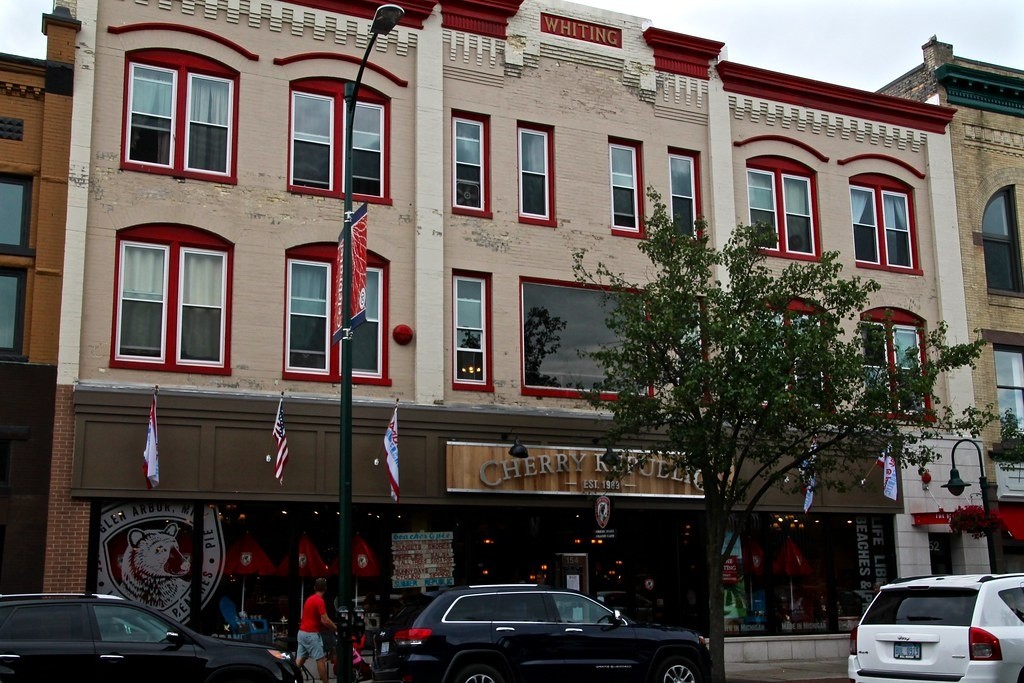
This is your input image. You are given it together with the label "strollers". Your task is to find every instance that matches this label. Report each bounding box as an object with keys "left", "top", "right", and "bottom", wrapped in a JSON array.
[{"left": 334, "top": 630, "right": 371, "bottom": 683}]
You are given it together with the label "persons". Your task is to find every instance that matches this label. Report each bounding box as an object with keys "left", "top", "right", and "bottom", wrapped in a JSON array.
[
  {"left": 331, "top": 534, "right": 380, "bottom": 607},
  {"left": 295, "top": 577, "right": 339, "bottom": 683}
]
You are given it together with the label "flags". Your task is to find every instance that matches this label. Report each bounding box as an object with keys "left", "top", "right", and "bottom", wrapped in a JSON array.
[
  {"left": 272, "top": 402, "right": 290, "bottom": 485},
  {"left": 142, "top": 397, "right": 160, "bottom": 489},
  {"left": 878, "top": 439, "right": 898, "bottom": 502},
  {"left": 798, "top": 441, "right": 819, "bottom": 517},
  {"left": 380, "top": 407, "right": 400, "bottom": 502}
]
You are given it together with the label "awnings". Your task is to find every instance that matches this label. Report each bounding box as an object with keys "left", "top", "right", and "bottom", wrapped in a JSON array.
[{"left": 986, "top": 504, "right": 1024, "bottom": 542}]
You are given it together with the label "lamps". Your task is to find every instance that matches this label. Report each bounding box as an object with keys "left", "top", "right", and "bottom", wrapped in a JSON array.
[
  {"left": 463, "top": 365, "right": 481, "bottom": 374},
  {"left": 502, "top": 428, "right": 529, "bottom": 458}
]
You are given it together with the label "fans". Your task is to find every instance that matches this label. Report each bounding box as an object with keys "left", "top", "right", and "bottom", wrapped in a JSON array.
[{"left": 456, "top": 182, "right": 480, "bottom": 208}]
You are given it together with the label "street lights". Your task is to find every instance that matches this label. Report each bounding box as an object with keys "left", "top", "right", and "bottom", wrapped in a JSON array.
[
  {"left": 339, "top": 4, "right": 406, "bottom": 622},
  {"left": 940, "top": 440, "right": 998, "bottom": 574}
]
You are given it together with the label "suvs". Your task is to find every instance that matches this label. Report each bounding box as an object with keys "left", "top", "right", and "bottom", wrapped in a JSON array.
[
  {"left": 370, "top": 584, "right": 712, "bottom": 683},
  {"left": 0, "top": 594, "right": 302, "bottom": 683},
  {"left": 846, "top": 573, "right": 1024, "bottom": 683}
]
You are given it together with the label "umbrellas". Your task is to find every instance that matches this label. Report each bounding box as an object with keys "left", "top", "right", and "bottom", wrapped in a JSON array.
[
  {"left": 274, "top": 535, "right": 332, "bottom": 620},
  {"left": 772, "top": 536, "right": 814, "bottom": 610},
  {"left": 211, "top": 533, "right": 278, "bottom": 618},
  {"left": 740, "top": 536, "right": 767, "bottom": 612}
]
